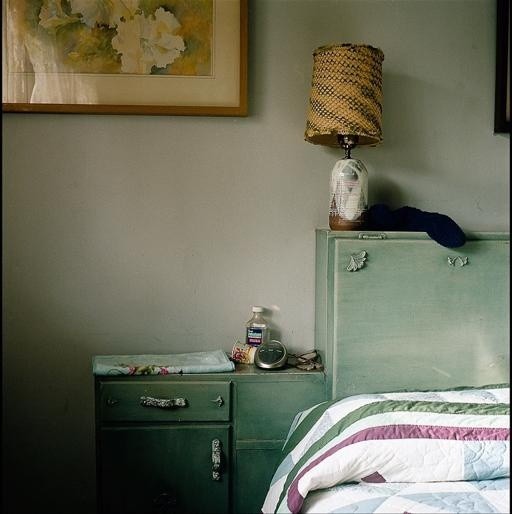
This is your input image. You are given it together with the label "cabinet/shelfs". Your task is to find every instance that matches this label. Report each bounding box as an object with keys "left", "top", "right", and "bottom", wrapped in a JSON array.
[{"left": 88, "top": 350, "right": 325, "bottom": 513}]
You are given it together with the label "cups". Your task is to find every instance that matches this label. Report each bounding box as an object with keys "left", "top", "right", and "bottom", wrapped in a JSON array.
[{"left": 231, "top": 340, "right": 256, "bottom": 365}]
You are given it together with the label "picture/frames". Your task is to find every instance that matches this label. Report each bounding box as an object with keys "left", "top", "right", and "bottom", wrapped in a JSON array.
[{"left": 1, "top": 0, "right": 249, "bottom": 118}]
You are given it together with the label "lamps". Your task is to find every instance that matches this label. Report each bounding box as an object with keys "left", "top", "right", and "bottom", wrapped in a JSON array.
[{"left": 304, "top": 41, "right": 385, "bottom": 232}]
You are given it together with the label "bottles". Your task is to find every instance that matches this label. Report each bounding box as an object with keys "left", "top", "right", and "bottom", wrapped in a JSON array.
[{"left": 246, "top": 306, "right": 270, "bottom": 347}]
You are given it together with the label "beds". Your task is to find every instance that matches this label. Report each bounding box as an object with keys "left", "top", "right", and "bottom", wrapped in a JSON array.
[{"left": 304, "top": 225, "right": 511, "bottom": 512}]
